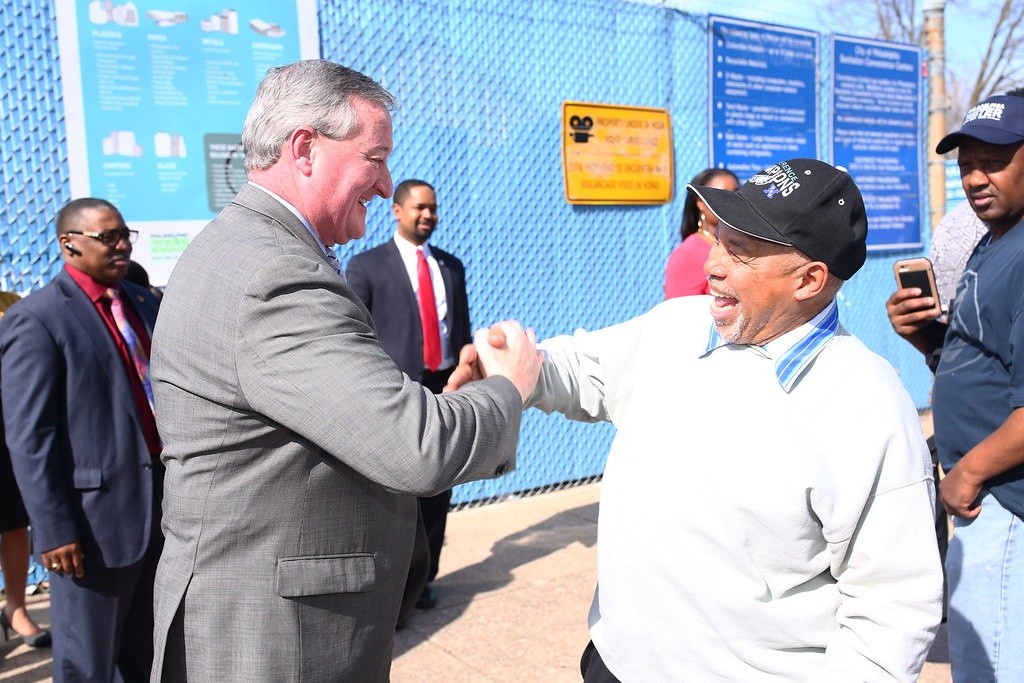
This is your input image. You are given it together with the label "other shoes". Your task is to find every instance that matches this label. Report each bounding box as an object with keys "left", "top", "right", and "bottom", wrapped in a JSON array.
[{"left": 415, "top": 582, "right": 441, "bottom": 609}]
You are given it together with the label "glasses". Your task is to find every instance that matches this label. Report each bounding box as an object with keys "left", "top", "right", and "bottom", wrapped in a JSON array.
[{"left": 64, "top": 228, "right": 139, "bottom": 247}]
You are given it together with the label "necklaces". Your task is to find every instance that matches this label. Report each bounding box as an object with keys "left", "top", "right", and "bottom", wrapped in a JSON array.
[{"left": 698, "top": 229, "right": 715, "bottom": 242}]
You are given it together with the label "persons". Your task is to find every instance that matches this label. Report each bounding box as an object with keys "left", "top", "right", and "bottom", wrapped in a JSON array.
[
  {"left": 149, "top": 61, "right": 544, "bottom": 683},
  {"left": 663, "top": 169, "right": 739, "bottom": 300},
  {"left": 345, "top": 179, "right": 472, "bottom": 610},
  {"left": 0, "top": 197, "right": 162, "bottom": 683},
  {"left": 886, "top": 88, "right": 1024, "bottom": 683},
  {"left": 444, "top": 159, "right": 944, "bottom": 683}
]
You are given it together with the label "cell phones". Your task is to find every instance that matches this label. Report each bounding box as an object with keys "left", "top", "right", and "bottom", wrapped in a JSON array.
[{"left": 894, "top": 258, "right": 942, "bottom": 322}]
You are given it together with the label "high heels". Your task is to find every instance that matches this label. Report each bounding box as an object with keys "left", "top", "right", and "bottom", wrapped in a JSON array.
[{"left": 0, "top": 607, "right": 53, "bottom": 647}]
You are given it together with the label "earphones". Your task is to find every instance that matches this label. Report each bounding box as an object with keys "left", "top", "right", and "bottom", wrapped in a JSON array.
[{"left": 65, "top": 243, "right": 81, "bottom": 257}]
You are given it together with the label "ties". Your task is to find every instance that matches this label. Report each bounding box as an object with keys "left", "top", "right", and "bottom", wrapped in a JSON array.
[
  {"left": 100, "top": 287, "right": 156, "bottom": 420},
  {"left": 416, "top": 249, "right": 443, "bottom": 373}
]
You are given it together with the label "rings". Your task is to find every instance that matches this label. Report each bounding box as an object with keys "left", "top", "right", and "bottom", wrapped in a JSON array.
[{"left": 52, "top": 563, "right": 61, "bottom": 571}]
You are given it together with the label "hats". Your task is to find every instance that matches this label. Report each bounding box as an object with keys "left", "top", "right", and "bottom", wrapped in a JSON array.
[
  {"left": 935, "top": 95, "right": 1024, "bottom": 154},
  {"left": 686, "top": 158, "right": 868, "bottom": 280}
]
